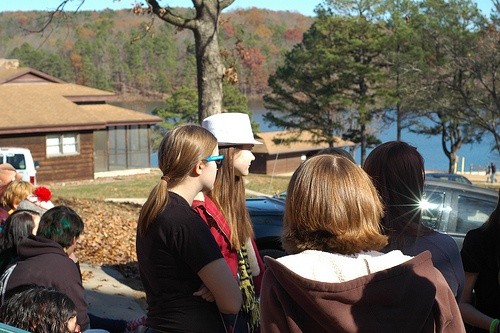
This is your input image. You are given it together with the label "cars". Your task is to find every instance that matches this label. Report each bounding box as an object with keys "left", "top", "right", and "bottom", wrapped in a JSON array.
[
  {"left": 427, "top": 173, "right": 472, "bottom": 185},
  {"left": 242, "top": 191, "right": 288, "bottom": 270},
  {"left": 421, "top": 181, "right": 499, "bottom": 255}
]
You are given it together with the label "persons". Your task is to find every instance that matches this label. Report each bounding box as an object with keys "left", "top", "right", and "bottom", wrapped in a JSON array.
[
  {"left": 0, "top": 112, "right": 500, "bottom": 333},
  {"left": 260, "top": 154, "right": 465, "bottom": 333}
]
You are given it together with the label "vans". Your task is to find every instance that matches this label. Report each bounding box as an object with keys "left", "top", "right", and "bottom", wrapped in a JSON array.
[{"left": 0, "top": 146, "right": 39, "bottom": 186}]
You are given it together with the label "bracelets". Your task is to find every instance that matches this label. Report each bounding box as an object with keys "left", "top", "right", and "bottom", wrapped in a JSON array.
[{"left": 490, "top": 319, "right": 500, "bottom": 332}]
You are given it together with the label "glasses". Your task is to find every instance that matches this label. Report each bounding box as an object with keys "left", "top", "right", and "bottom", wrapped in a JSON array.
[{"left": 201, "top": 154, "right": 224, "bottom": 168}]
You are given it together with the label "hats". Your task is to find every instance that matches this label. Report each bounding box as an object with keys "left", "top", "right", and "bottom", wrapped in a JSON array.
[
  {"left": 17, "top": 186, "right": 55, "bottom": 217},
  {"left": 202, "top": 112, "right": 263, "bottom": 145}
]
